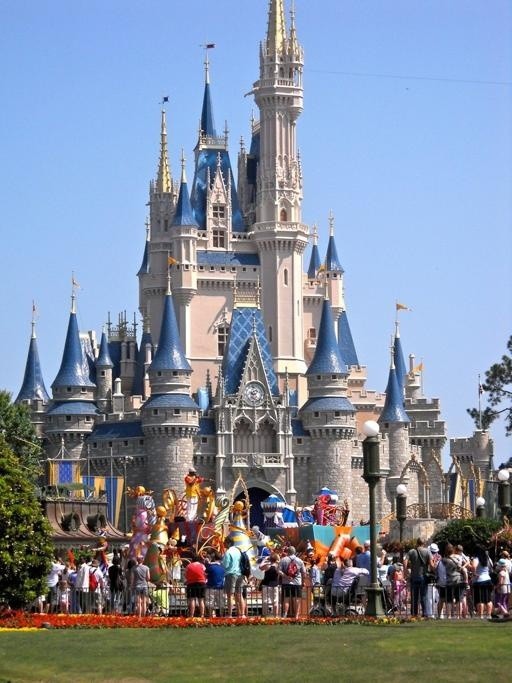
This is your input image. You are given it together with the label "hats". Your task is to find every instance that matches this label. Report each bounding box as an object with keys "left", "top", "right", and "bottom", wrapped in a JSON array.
[
  {"left": 427, "top": 543, "right": 440, "bottom": 553},
  {"left": 88, "top": 560, "right": 100, "bottom": 566}
]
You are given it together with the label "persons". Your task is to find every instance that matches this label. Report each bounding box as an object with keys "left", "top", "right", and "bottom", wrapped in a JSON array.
[{"left": 29, "top": 537, "right": 511, "bottom": 620}]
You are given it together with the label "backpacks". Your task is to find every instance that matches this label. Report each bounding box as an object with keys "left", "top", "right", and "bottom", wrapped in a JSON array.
[
  {"left": 235, "top": 546, "right": 250, "bottom": 578},
  {"left": 286, "top": 556, "right": 299, "bottom": 577},
  {"left": 89, "top": 569, "right": 98, "bottom": 591}
]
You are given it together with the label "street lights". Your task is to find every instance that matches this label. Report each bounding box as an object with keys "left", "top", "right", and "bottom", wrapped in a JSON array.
[
  {"left": 360, "top": 420, "right": 407, "bottom": 618},
  {"left": 498, "top": 470, "right": 510, "bottom": 528},
  {"left": 117, "top": 455, "right": 133, "bottom": 533},
  {"left": 476, "top": 497, "right": 485, "bottom": 517}
]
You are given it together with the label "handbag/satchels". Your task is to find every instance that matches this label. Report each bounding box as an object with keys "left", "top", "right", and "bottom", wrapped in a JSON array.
[
  {"left": 489, "top": 570, "right": 500, "bottom": 586},
  {"left": 116, "top": 575, "right": 128, "bottom": 593}
]
